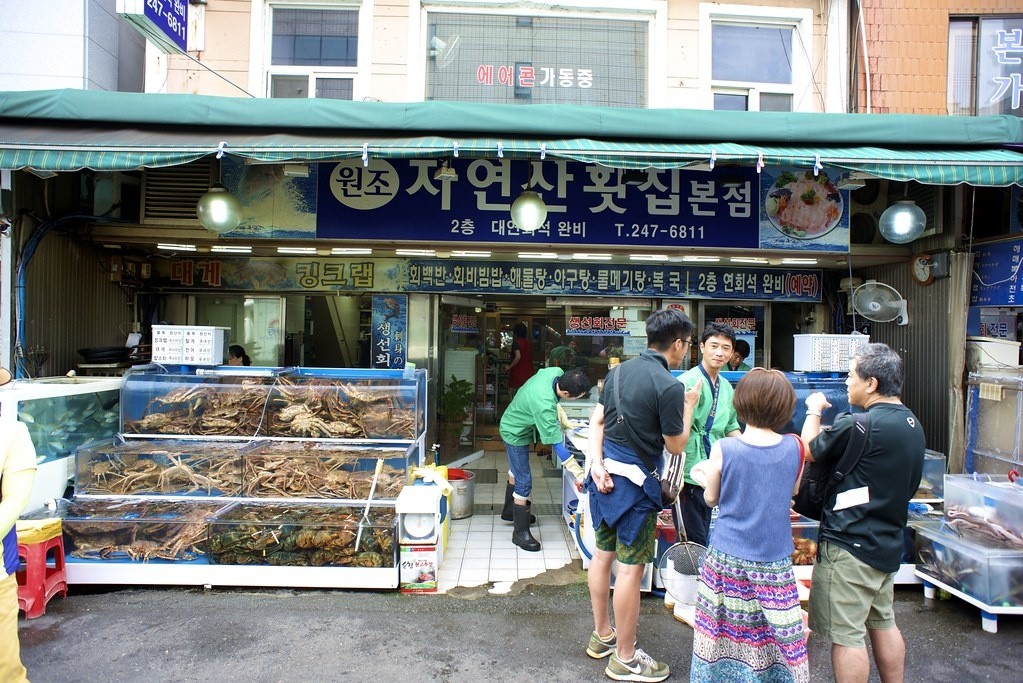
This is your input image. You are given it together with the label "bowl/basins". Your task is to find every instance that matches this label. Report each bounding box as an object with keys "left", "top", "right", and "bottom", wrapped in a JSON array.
[{"left": 404, "top": 513, "right": 435, "bottom": 538}]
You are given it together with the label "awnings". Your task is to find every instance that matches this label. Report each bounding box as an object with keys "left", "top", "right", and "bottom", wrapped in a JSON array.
[{"left": 0, "top": 91, "right": 1023, "bottom": 187}]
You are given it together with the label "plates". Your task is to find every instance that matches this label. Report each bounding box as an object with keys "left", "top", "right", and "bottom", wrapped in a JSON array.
[{"left": 765, "top": 172, "right": 843, "bottom": 239}]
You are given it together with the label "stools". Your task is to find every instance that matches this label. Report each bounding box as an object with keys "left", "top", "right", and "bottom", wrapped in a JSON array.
[{"left": 15, "top": 518, "right": 68, "bottom": 621}]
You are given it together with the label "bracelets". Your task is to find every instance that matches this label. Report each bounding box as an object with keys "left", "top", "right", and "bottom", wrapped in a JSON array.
[{"left": 805, "top": 410, "right": 823, "bottom": 417}]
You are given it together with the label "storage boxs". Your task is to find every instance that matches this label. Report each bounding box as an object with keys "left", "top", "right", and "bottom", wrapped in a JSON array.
[
  {"left": 943, "top": 474, "right": 1023, "bottom": 539},
  {"left": 790, "top": 515, "right": 821, "bottom": 566},
  {"left": 908, "top": 449, "right": 947, "bottom": 503},
  {"left": 911, "top": 521, "right": 1023, "bottom": 607},
  {"left": 966, "top": 336, "right": 1021, "bottom": 371},
  {"left": 19, "top": 369, "right": 427, "bottom": 569}
]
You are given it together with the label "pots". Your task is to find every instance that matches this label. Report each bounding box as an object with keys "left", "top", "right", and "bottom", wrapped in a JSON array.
[{"left": 77, "top": 344, "right": 152, "bottom": 364}]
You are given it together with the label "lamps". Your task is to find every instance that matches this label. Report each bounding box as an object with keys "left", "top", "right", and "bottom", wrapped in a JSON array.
[
  {"left": 196, "top": 157, "right": 244, "bottom": 235},
  {"left": 434, "top": 157, "right": 456, "bottom": 181},
  {"left": 879, "top": 181, "right": 926, "bottom": 244},
  {"left": 510, "top": 161, "right": 547, "bottom": 231},
  {"left": 836, "top": 171, "right": 866, "bottom": 190},
  {"left": 282, "top": 164, "right": 312, "bottom": 178}
]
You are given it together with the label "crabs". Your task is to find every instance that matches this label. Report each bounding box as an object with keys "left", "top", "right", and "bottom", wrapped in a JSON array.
[{"left": 55, "top": 370, "right": 422, "bottom": 570}]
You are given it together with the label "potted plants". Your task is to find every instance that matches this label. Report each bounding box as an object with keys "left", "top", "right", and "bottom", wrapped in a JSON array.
[{"left": 437, "top": 374, "right": 483, "bottom": 464}]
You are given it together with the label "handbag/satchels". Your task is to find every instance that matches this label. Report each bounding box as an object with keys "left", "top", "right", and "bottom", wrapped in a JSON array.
[{"left": 659, "top": 444, "right": 686, "bottom": 508}]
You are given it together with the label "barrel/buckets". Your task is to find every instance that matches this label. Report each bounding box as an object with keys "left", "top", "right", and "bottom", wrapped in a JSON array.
[{"left": 448, "top": 469, "right": 476, "bottom": 519}]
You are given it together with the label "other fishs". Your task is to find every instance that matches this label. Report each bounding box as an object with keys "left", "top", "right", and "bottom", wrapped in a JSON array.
[{"left": 919, "top": 499, "right": 1023, "bottom": 606}]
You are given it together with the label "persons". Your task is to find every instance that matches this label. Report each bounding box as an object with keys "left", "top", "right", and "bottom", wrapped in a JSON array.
[
  {"left": 793, "top": 344, "right": 926, "bottom": 683},
  {"left": 721, "top": 338, "right": 751, "bottom": 371},
  {"left": 0, "top": 365, "right": 39, "bottom": 683},
  {"left": 501, "top": 367, "right": 585, "bottom": 552},
  {"left": 227, "top": 343, "right": 252, "bottom": 369},
  {"left": 502, "top": 323, "right": 534, "bottom": 405},
  {"left": 664, "top": 322, "right": 743, "bottom": 630},
  {"left": 689, "top": 370, "right": 811, "bottom": 683},
  {"left": 586, "top": 308, "right": 703, "bottom": 683},
  {"left": 551, "top": 325, "right": 585, "bottom": 365}
]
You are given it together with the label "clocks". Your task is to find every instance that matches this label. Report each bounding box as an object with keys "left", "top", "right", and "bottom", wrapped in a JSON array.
[{"left": 910, "top": 254, "right": 935, "bottom": 287}]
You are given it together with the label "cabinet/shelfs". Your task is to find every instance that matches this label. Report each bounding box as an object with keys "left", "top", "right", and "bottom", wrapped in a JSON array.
[{"left": 476, "top": 358, "right": 545, "bottom": 423}]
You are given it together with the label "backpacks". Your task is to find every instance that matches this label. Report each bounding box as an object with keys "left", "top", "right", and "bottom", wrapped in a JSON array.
[{"left": 793, "top": 411, "right": 870, "bottom": 563}]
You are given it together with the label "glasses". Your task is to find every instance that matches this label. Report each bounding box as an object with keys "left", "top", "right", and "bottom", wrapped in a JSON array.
[
  {"left": 671, "top": 337, "right": 692, "bottom": 348},
  {"left": 746, "top": 366, "right": 785, "bottom": 379}
]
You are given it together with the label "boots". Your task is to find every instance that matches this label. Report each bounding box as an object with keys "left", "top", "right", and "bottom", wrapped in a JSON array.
[
  {"left": 500, "top": 480, "right": 536, "bottom": 524},
  {"left": 512, "top": 500, "right": 541, "bottom": 552},
  {"left": 664, "top": 556, "right": 676, "bottom": 608},
  {"left": 673, "top": 568, "right": 701, "bottom": 629}
]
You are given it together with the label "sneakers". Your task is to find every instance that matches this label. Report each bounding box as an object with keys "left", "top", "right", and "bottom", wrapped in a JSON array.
[
  {"left": 587, "top": 627, "right": 638, "bottom": 659},
  {"left": 605, "top": 649, "right": 670, "bottom": 682}
]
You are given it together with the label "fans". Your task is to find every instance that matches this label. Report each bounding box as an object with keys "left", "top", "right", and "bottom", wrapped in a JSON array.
[
  {"left": 429, "top": 34, "right": 461, "bottom": 69},
  {"left": 853, "top": 281, "right": 908, "bottom": 326}
]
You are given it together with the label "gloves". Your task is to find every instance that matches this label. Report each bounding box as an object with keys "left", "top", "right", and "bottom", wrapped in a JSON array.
[
  {"left": 561, "top": 454, "right": 585, "bottom": 483},
  {"left": 556, "top": 402, "right": 576, "bottom": 429}
]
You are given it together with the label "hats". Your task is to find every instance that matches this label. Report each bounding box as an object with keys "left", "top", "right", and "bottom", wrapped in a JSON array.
[{"left": 505, "top": 321, "right": 527, "bottom": 336}]
